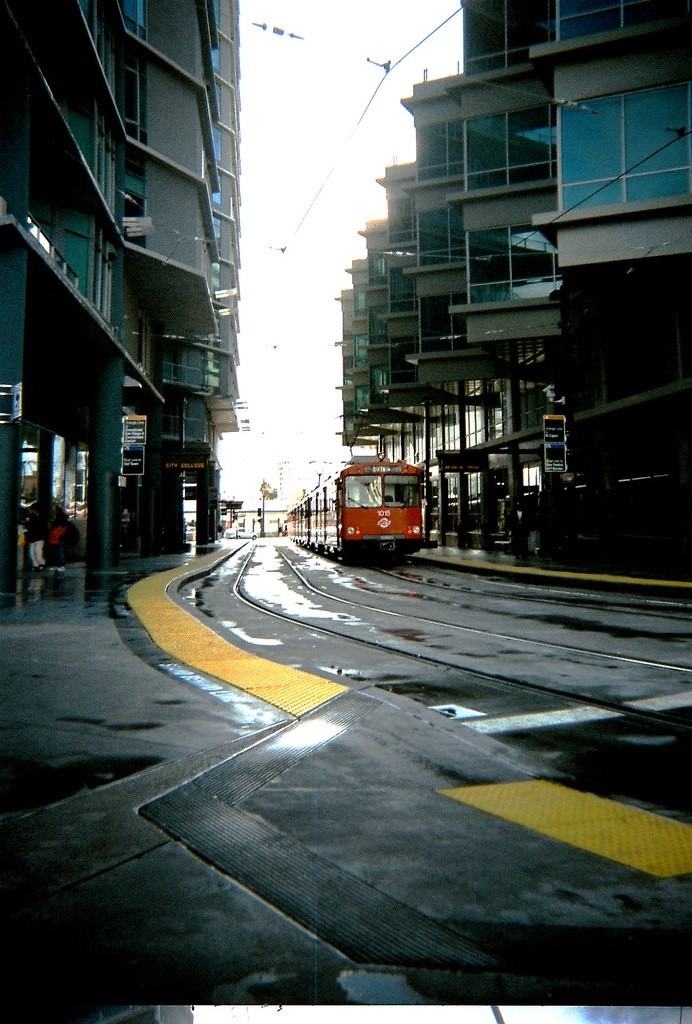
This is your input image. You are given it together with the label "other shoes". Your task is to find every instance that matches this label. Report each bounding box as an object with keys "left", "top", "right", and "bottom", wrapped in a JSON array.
[
  {"left": 58, "top": 567, "right": 65, "bottom": 572},
  {"left": 49, "top": 566, "right": 58, "bottom": 571},
  {"left": 32, "top": 565, "right": 45, "bottom": 571}
]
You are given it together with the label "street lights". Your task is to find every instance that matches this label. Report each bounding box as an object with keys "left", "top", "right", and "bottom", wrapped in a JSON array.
[{"left": 259, "top": 494, "right": 268, "bottom": 537}]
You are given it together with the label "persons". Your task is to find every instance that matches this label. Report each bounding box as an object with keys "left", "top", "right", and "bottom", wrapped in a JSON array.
[
  {"left": 236, "top": 527, "right": 239, "bottom": 539},
  {"left": 217, "top": 522, "right": 223, "bottom": 542},
  {"left": 278, "top": 525, "right": 288, "bottom": 538},
  {"left": 184, "top": 525, "right": 187, "bottom": 542},
  {"left": 24, "top": 502, "right": 72, "bottom": 572},
  {"left": 505, "top": 501, "right": 559, "bottom": 562}
]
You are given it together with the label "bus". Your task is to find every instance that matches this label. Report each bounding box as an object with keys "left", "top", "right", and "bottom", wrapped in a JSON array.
[{"left": 284, "top": 412, "right": 425, "bottom": 564}]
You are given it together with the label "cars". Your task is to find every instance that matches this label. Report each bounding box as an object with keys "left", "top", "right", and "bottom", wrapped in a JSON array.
[{"left": 224, "top": 526, "right": 258, "bottom": 540}]
